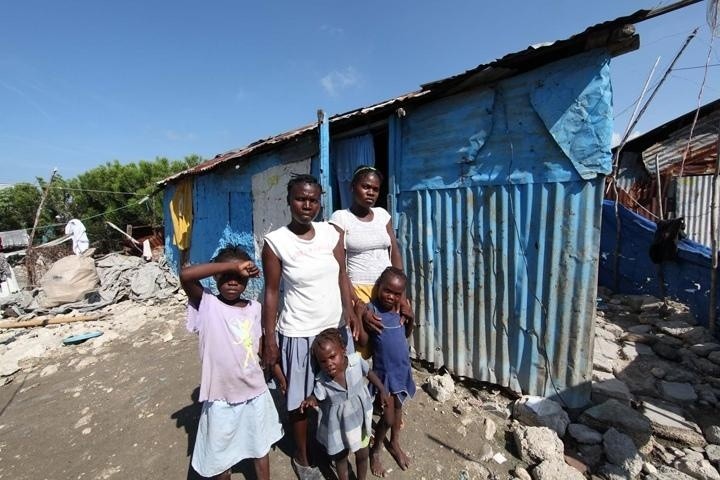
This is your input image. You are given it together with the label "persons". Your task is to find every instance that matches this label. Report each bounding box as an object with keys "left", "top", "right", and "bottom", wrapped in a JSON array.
[
  {"left": 299, "top": 328, "right": 391, "bottom": 480},
  {"left": 327, "top": 165, "right": 409, "bottom": 447},
  {"left": 180, "top": 243, "right": 287, "bottom": 479},
  {"left": 354, "top": 267, "right": 415, "bottom": 477},
  {"left": 260, "top": 174, "right": 362, "bottom": 479}
]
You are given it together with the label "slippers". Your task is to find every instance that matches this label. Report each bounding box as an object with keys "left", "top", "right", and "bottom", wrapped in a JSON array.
[{"left": 293, "top": 458, "right": 326, "bottom": 480}]
